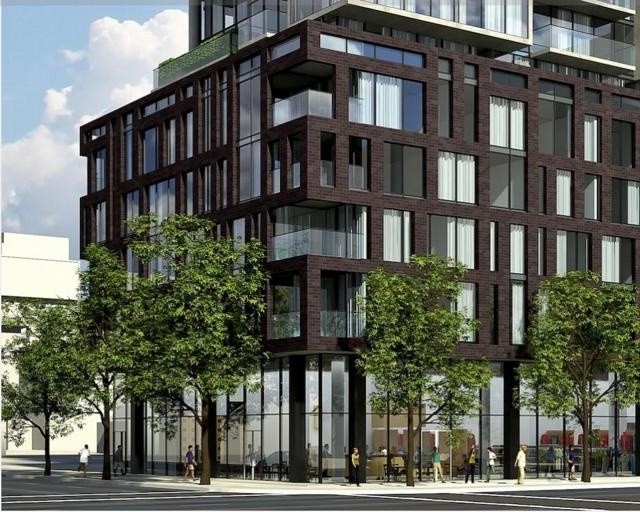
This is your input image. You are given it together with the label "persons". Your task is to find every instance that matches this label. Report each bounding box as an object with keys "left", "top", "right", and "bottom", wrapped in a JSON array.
[
  {"left": 431, "top": 446, "right": 446, "bottom": 483},
  {"left": 182, "top": 443, "right": 200, "bottom": 481},
  {"left": 567, "top": 443, "right": 577, "bottom": 480},
  {"left": 113, "top": 445, "right": 128, "bottom": 475},
  {"left": 514, "top": 444, "right": 529, "bottom": 484},
  {"left": 245, "top": 443, "right": 258, "bottom": 479},
  {"left": 465, "top": 444, "right": 478, "bottom": 483},
  {"left": 77, "top": 443, "right": 90, "bottom": 477},
  {"left": 322, "top": 443, "right": 333, "bottom": 477},
  {"left": 305, "top": 443, "right": 314, "bottom": 480},
  {"left": 484, "top": 446, "right": 497, "bottom": 482},
  {"left": 543, "top": 445, "right": 557, "bottom": 478},
  {"left": 348, "top": 447, "right": 360, "bottom": 486},
  {"left": 366, "top": 444, "right": 422, "bottom": 480},
  {"left": 457, "top": 453, "right": 470, "bottom": 473},
  {"left": 194, "top": 443, "right": 202, "bottom": 480}
]
